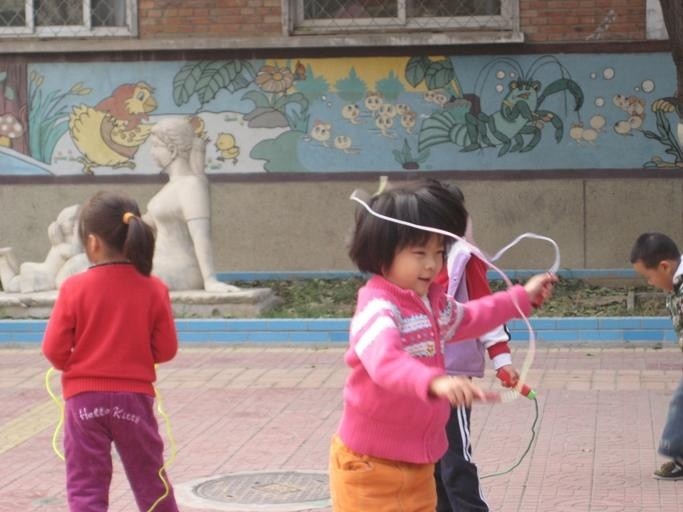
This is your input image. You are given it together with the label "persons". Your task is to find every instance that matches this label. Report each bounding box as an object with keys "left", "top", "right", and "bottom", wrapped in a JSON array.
[
  {"left": 328, "top": 190, "right": 561, "bottom": 512},
  {"left": 0, "top": 109, "right": 240, "bottom": 293},
  {"left": 628, "top": 232, "right": 683, "bottom": 481},
  {"left": 41, "top": 191, "right": 183, "bottom": 512},
  {"left": 10, "top": 203, "right": 85, "bottom": 293},
  {"left": 415, "top": 174, "right": 525, "bottom": 512}
]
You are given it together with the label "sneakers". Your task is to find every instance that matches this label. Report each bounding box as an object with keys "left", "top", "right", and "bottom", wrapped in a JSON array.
[{"left": 652, "top": 460, "right": 683, "bottom": 481}]
